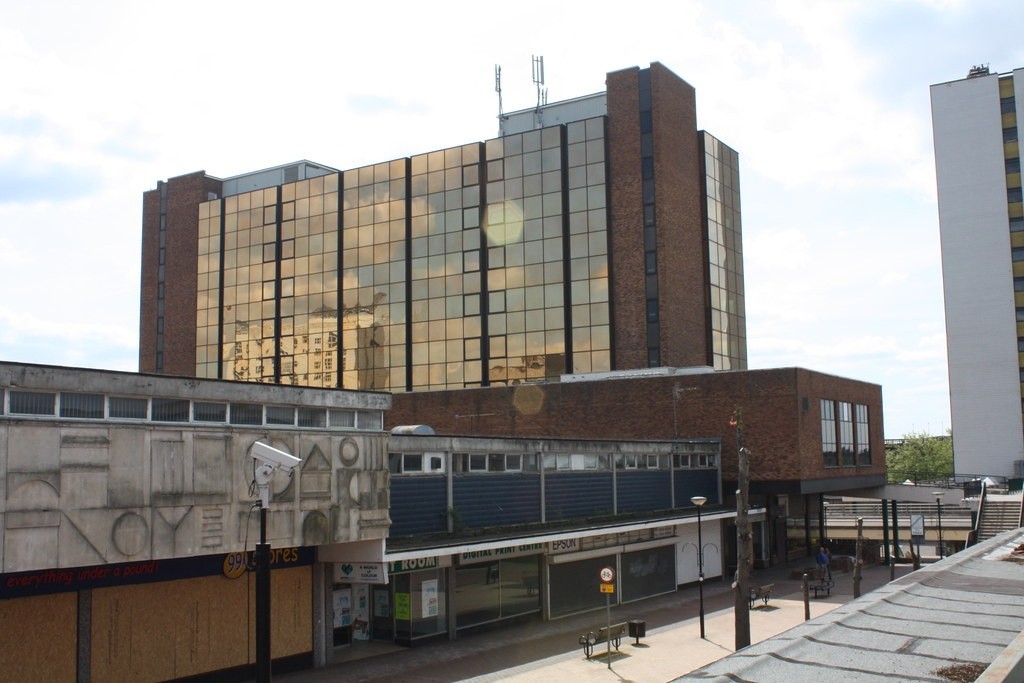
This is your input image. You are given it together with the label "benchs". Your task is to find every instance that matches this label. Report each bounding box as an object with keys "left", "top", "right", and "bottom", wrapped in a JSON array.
[
  {"left": 749, "top": 583, "right": 776, "bottom": 609},
  {"left": 810, "top": 578, "right": 836, "bottom": 598},
  {"left": 579, "top": 622, "right": 627, "bottom": 658}
]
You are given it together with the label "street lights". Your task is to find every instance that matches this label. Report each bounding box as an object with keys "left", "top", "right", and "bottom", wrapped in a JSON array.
[
  {"left": 932, "top": 491, "right": 945, "bottom": 559},
  {"left": 690, "top": 496, "right": 707, "bottom": 639}
]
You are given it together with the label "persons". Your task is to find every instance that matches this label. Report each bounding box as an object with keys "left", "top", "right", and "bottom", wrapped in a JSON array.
[
  {"left": 816, "top": 547, "right": 832, "bottom": 580},
  {"left": 947, "top": 549, "right": 952, "bottom": 557}
]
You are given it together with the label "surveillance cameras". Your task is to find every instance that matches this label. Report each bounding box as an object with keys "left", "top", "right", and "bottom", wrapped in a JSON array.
[{"left": 251, "top": 440, "right": 302, "bottom": 473}]
